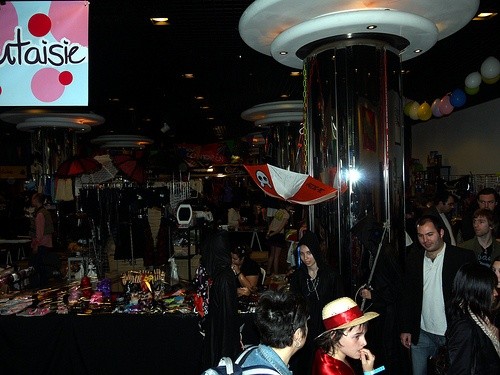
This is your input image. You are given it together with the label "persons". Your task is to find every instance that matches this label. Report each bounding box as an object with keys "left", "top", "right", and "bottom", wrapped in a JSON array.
[{"left": 29, "top": 189, "right": 500, "bottom": 375}]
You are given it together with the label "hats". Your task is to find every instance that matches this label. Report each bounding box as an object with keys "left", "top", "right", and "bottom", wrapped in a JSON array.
[{"left": 314, "top": 296, "right": 380, "bottom": 341}]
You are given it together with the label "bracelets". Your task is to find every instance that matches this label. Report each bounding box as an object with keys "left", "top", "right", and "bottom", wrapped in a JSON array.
[
  {"left": 237, "top": 272, "right": 242, "bottom": 277},
  {"left": 363, "top": 365, "right": 385, "bottom": 375}
]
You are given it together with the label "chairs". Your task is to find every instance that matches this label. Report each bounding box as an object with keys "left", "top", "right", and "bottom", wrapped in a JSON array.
[{"left": 258, "top": 267, "right": 267, "bottom": 286}]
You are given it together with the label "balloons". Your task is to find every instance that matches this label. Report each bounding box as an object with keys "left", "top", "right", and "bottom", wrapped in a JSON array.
[{"left": 404, "top": 56, "right": 500, "bottom": 120}]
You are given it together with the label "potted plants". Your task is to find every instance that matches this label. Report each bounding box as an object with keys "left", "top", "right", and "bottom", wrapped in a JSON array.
[{"left": 414, "top": 163, "right": 423, "bottom": 180}]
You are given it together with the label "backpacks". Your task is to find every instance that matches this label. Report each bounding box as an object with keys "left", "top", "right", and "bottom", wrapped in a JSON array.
[{"left": 202, "top": 345, "right": 281, "bottom": 375}]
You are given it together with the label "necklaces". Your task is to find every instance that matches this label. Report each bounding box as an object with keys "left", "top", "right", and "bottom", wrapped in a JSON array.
[
  {"left": 307, "top": 277, "right": 319, "bottom": 292},
  {"left": 308, "top": 279, "right": 320, "bottom": 300}
]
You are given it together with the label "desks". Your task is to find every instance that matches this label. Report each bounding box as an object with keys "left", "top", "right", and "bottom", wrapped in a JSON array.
[
  {"left": 0, "top": 281, "right": 215, "bottom": 375},
  {"left": 201, "top": 225, "right": 269, "bottom": 281}
]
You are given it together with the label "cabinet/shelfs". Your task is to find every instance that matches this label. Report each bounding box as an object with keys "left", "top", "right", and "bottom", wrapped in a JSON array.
[
  {"left": 170, "top": 224, "right": 198, "bottom": 279},
  {"left": 427, "top": 166, "right": 451, "bottom": 182}
]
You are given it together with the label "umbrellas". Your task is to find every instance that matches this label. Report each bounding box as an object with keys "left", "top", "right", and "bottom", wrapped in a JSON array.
[
  {"left": 58, "top": 154, "right": 147, "bottom": 184},
  {"left": 241, "top": 163, "right": 339, "bottom": 205}
]
